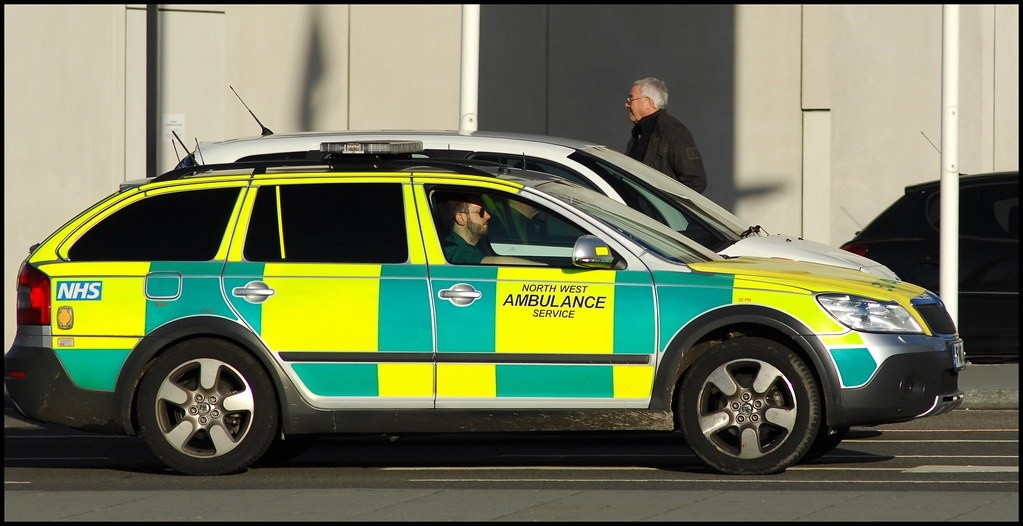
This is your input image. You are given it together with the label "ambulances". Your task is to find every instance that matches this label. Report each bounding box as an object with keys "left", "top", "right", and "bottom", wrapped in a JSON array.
[{"left": 5, "top": 154, "right": 965, "bottom": 475}]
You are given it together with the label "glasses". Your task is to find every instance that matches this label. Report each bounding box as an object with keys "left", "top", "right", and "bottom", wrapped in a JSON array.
[
  {"left": 459, "top": 207, "right": 485, "bottom": 218},
  {"left": 624, "top": 97, "right": 642, "bottom": 104}
]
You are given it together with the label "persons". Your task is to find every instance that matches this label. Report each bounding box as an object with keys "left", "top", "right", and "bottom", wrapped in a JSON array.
[
  {"left": 627, "top": 77, "right": 708, "bottom": 194},
  {"left": 441, "top": 191, "right": 548, "bottom": 266}
]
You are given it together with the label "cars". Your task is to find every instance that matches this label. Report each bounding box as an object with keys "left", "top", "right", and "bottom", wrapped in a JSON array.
[
  {"left": 837, "top": 172, "right": 1020, "bottom": 366},
  {"left": 120, "top": 129, "right": 899, "bottom": 281}
]
